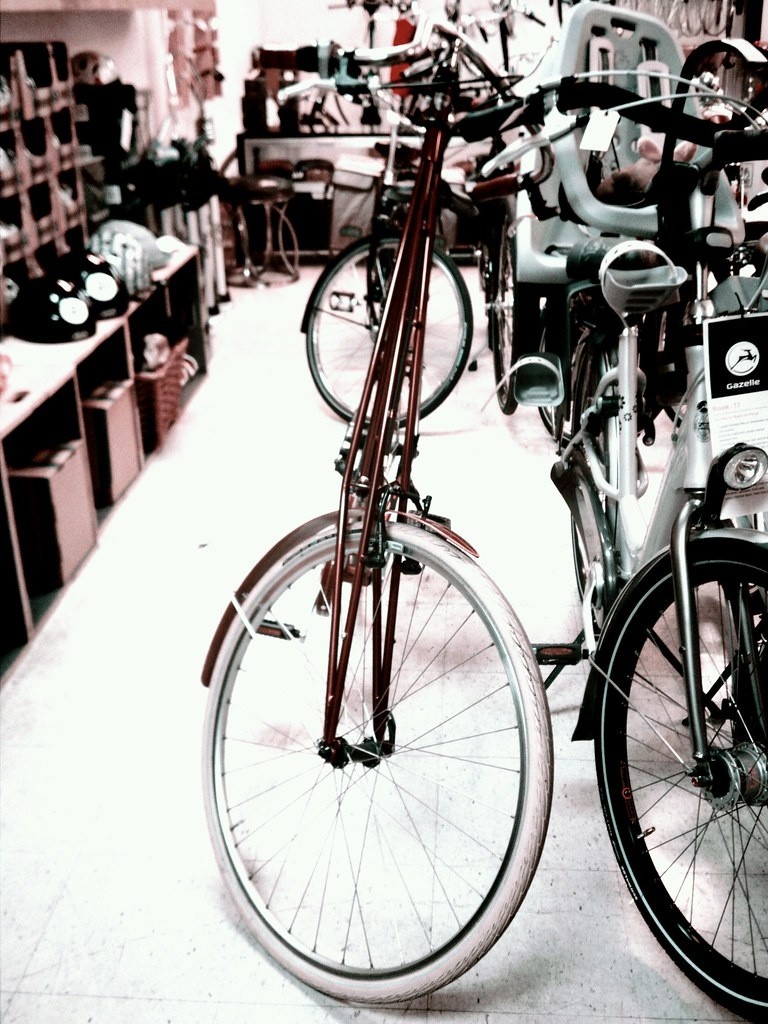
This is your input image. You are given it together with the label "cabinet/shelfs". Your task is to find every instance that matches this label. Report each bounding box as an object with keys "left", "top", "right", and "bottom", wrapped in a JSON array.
[
  {"left": 234, "top": 128, "right": 511, "bottom": 262},
  {"left": 0, "top": 246, "right": 206, "bottom": 695},
  {"left": 0, "top": 35, "right": 113, "bottom": 309}
]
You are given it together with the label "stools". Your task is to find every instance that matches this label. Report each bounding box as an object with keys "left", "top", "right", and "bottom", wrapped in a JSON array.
[{"left": 236, "top": 175, "right": 298, "bottom": 285}]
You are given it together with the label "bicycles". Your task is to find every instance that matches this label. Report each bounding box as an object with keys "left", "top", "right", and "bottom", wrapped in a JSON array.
[
  {"left": 523, "top": 35, "right": 768, "bottom": 1022},
  {"left": 197, "top": 18, "right": 558, "bottom": 1011},
  {"left": 296, "top": 34, "right": 527, "bottom": 434}
]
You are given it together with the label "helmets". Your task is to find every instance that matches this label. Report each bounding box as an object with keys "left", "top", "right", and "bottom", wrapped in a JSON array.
[
  {"left": 46, "top": 251, "right": 130, "bottom": 320},
  {"left": 4, "top": 276, "right": 97, "bottom": 343},
  {"left": 90, "top": 220, "right": 171, "bottom": 272}
]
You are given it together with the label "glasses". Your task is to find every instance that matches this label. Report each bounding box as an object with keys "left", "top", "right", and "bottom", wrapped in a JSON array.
[{"left": 81, "top": 63, "right": 99, "bottom": 75}]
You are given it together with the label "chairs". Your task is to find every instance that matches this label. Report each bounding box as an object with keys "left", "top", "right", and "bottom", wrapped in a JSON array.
[{"left": 515, "top": 0, "right": 746, "bottom": 281}]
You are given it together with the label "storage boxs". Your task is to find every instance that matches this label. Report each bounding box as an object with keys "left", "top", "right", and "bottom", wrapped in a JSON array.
[
  {"left": 11, "top": 439, "right": 97, "bottom": 591},
  {"left": 82, "top": 373, "right": 144, "bottom": 508},
  {"left": 136, "top": 337, "right": 189, "bottom": 448}
]
